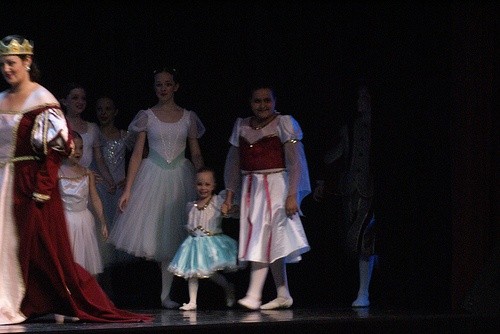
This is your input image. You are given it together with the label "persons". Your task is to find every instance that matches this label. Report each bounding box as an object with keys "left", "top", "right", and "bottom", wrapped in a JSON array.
[
  {"left": 0, "top": 34, "right": 312, "bottom": 325},
  {"left": 313, "top": 83, "right": 383, "bottom": 306}
]
